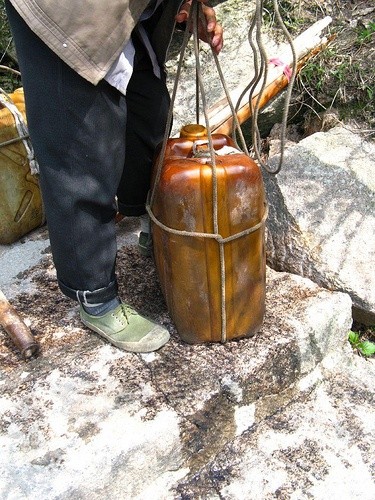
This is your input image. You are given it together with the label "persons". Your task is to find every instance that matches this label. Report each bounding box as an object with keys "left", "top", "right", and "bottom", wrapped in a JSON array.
[{"left": 6, "top": 0, "right": 223, "bottom": 352}]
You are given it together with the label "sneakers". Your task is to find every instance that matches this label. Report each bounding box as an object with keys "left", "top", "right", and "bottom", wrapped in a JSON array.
[{"left": 79, "top": 302, "right": 171, "bottom": 351}]
